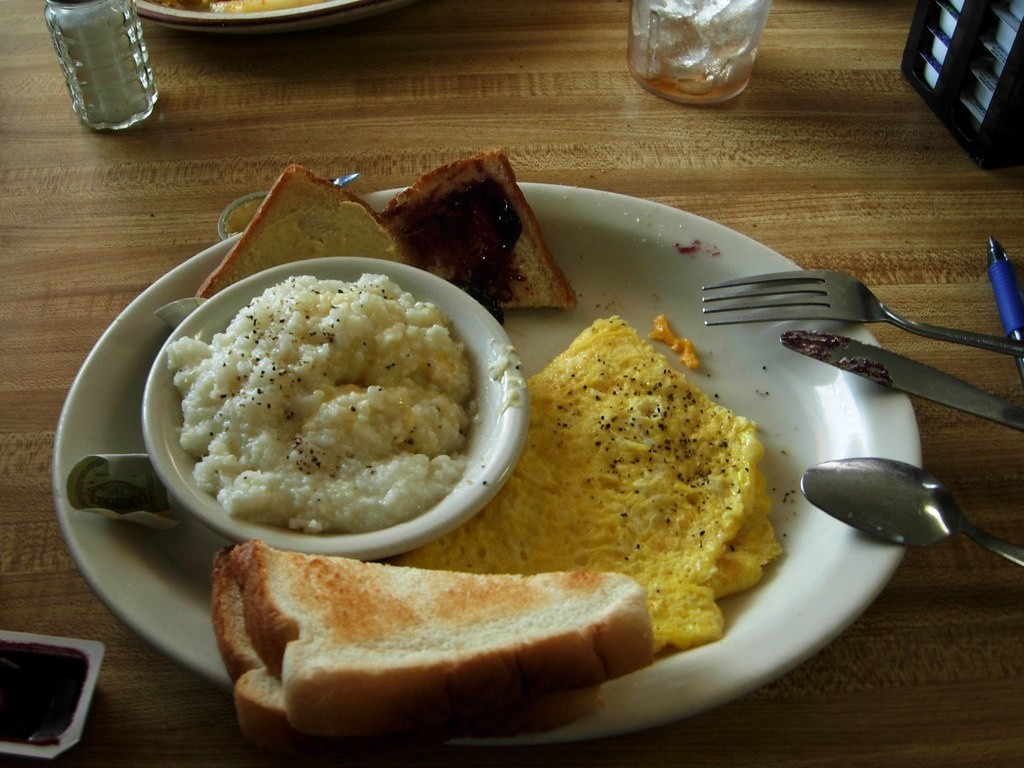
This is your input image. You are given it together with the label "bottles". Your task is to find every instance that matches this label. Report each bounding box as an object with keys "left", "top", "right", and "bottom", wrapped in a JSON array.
[{"left": 44, "top": 0, "right": 158, "bottom": 131}]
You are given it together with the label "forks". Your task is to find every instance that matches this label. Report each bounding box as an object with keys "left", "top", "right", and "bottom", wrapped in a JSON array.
[{"left": 701, "top": 268, "right": 1024, "bottom": 358}]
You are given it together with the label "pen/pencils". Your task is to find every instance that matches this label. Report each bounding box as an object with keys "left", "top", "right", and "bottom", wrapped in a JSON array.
[{"left": 985, "top": 232, "right": 1024, "bottom": 387}]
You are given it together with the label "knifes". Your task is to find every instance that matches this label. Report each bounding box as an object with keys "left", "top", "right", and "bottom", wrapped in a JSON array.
[{"left": 780, "top": 328, "right": 1024, "bottom": 432}]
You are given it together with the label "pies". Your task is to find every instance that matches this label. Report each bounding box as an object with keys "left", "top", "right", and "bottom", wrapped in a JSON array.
[{"left": 380, "top": 315, "right": 780, "bottom": 656}]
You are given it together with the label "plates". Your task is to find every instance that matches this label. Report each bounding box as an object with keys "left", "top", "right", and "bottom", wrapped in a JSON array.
[
  {"left": 51, "top": 176, "right": 923, "bottom": 748},
  {"left": 134, "top": 0, "right": 408, "bottom": 37}
]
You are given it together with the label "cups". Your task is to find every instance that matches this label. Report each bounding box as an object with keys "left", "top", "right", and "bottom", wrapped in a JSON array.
[{"left": 625, "top": 0, "right": 772, "bottom": 106}]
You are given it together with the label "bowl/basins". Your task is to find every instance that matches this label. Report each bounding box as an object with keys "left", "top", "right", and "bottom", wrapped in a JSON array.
[{"left": 142, "top": 253, "right": 530, "bottom": 560}]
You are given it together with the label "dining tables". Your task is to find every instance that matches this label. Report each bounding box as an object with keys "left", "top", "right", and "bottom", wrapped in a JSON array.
[{"left": 0, "top": 1, "right": 1024, "bottom": 768}]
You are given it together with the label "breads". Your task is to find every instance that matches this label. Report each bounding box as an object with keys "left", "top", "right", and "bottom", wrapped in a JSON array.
[
  {"left": 194, "top": 163, "right": 408, "bottom": 301},
  {"left": 208, "top": 537, "right": 657, "bottom": 751},
  {"left": 372, "top": 147, "right": 578, "bottom": 310}
]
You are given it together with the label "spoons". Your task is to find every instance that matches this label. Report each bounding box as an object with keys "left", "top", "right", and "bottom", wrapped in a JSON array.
[{"left": 800, "top": 457, "right": 1024, "bottom": 568}]
